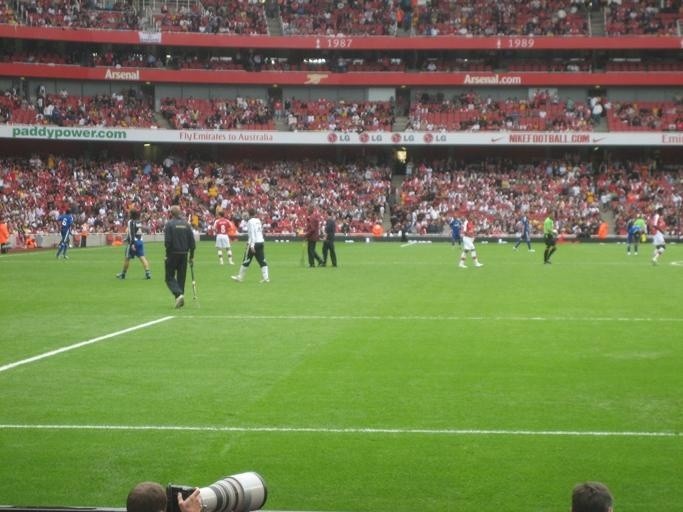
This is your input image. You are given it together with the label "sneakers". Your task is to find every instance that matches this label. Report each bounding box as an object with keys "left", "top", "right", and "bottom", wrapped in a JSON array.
[{"left": 175, "top": 295, "right": 183, "bottom": 309}]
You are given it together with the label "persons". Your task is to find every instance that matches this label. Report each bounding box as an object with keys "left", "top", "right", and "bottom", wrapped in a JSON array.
[
  {"left": 127, "top": 481, "right": 203, "bottom": 512},
  {"left": 570, "top": 482, "right": 614, "bottom": 512},
  {"left": 56, "top": 208, "right": 75, "bottom": 261},
  {"left": 650, "top": 207, "right": 666, "bottom": 265},
  {"left": 449, "top": 213, "right": 463, "bottom": 250},
  {"left": 512, "top": 212, "right": 535, "bottom": 253},
  {"left": 164, "top": 205, "right": 196, "bottom": 309},
  {"left": 322, "top": 210, "right": 337, "bottom": 266},
  {"left": 457, "top": 214, "right": 484, "bottom": 269},
  {"left": 230, "top": 207, "right": 269, "bottom": 283},
  {"left": 212, "top": 211, "right": 236, "bottom": 264},
  {"left": 2, "top": 2, "right": 683, "bottom": 240},
  {"left": 543, "top": 209, "right": 558, "bottom": 264},
  {"left": 305, "top": 206, "right": 324, "bottom": 267},
  {"left": 627, "top": 225, "right": 641, "bottom": 255},
  {"left": 115, "top": 207, "right": 152, "bottom": 278}
]
